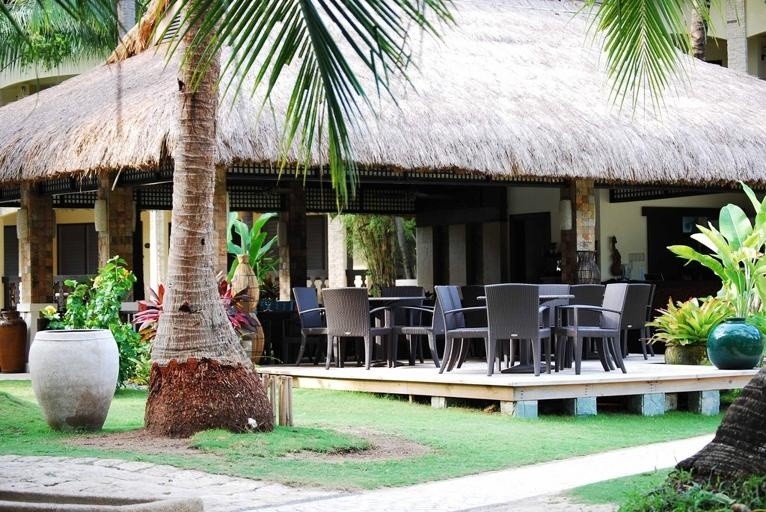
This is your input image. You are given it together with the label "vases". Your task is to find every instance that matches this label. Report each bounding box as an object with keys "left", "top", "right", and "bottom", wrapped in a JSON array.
[
  {"left": 0, "top": 311, "right": 27, "bottom": 373},
  {"left": 28, "top": 329, "right": 120, "bottom": 433}
]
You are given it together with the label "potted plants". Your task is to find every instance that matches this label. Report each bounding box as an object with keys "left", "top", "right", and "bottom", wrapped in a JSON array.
[
  {"left": 666, "top": 179, "right": 766, "bottom": 369},
  {"left": 638, "top": 294, "right": 731, "bottom": 364}
]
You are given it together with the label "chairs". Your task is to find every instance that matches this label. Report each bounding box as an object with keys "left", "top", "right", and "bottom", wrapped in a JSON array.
[
  {"left": 434, "top": 285, "right": 501, "bottom": 374},
  {"left": 292, "top": 285, "right": 338, "bottom": 367},
  {"left": 569, "top": 284, "right": 605, "bottom": 354},
  {"left": 620, "top": 283, "right": 651, "bottom": 359},
  {"left": 645, "top": 286, "right": 656, "bottom": 357},
  {"left": 320, "top": 287, "right": 393, "bottom": 371},
  {"left": 393, "top": 296, "right": 446, "bottom": 368},
  {"left": 460, "top": 282, "right": 488, "bottom": 360},
  {"left": 556, "top": 282, "right": 629, "bottom": 373},
  {"left": 540, "top": 284, "right": 570, "bottom": 329},
  {"left": 484, "top": 283, "right": 551, "bottom": 376},
  {"left": 379, "top": 285, "right": 426, "bottom": 364}
]
